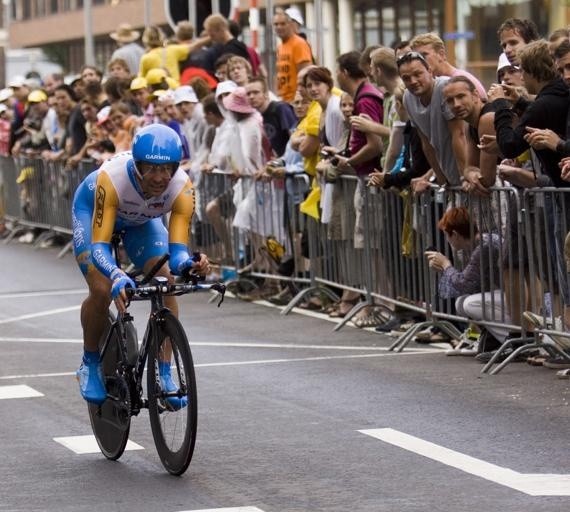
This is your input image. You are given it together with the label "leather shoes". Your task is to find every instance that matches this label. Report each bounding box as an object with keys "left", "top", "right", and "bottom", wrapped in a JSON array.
[{"left": 374, "top": 313, "right": 406, "bottom": 331}]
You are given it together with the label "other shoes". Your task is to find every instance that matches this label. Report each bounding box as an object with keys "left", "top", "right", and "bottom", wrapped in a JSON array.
[
  {"left": 269, "top": 290, "right": 293, "bottom": 306},
  {"left": 238, "top": 284, "right": 278, "bottom": 301}
]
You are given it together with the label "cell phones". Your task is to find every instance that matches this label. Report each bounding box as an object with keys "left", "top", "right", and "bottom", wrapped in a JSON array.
[{"left": 426, "top": 246, "right": 438, "bottom": 252}]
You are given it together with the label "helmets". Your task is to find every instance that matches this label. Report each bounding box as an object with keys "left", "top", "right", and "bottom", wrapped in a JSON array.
[{"left": 130, "top": 122, "right": 185, "bottom": 182}]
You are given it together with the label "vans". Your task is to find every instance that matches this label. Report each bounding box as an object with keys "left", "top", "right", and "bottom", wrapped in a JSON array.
[{"left": 4, "top": 47, "right": 70, "bottom": 92}]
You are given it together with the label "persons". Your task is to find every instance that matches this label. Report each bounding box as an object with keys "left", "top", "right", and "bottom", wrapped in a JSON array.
[
  {"left": 0, "top": 0, "right": 569, "bottom": 380},
  {"left": 75, "top": 122, "right": 210, "bottom": 413}
]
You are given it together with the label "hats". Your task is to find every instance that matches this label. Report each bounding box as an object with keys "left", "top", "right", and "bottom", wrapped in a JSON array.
[
  {"left": 108, "top": 24, "right": 141, "bottom": 44},
  {"left": 15, "top": 166, "right": 37, "bottom": 186},
  {"left": 128, "top": 65, "right": 256, "bottom": 117},
  {"left": 495, "top": 51, "right": 522, "bottom": 85},
  {"left": 95, "top": 104, "right": 113, "bottom": 127},
  {"left": 0, "top": 74, "right": 49, "bottom": 119},
  {"left": 284, "top": 6, "right": 304, "bottom": 28}
]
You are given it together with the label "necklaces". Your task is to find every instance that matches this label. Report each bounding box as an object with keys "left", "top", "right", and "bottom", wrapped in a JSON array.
[{"left": 140, "top": 191, "right": 153, "bottom": 210}]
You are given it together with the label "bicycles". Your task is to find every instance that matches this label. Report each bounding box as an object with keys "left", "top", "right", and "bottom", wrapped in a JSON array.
[{"left": 81, "top": 250, "right": 234, "bottom": 481}]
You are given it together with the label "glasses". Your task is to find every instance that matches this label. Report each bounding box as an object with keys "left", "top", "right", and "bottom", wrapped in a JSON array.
[{"left": 395, "top": 52, "right": 430, "bottom": 70}]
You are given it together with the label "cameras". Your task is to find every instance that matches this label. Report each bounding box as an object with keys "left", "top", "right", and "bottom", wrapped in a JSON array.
[{"left": 319, "top": 150, "right": 340, "bottom": 167}]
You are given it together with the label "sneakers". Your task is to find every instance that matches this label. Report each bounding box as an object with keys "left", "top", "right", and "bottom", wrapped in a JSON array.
[
  {"left": 522, "top": 309, "right": 570, "bottom": 363},
  {"left": 74, "top": 361, "right": 108, "bottom": 405},
  {"left": 152, "top": 376, "right": 191, "bottom": 413},
  {"left": 392, "top": 321, "right": 570, "bottom": 380},
  {"left": 1, "top": 220, "right": 76, "bottom": 251}
]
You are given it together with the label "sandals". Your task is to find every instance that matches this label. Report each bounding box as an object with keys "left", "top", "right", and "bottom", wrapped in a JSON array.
[{"left": 298, "top": 289, "right": 396, "bottom": 328}]
